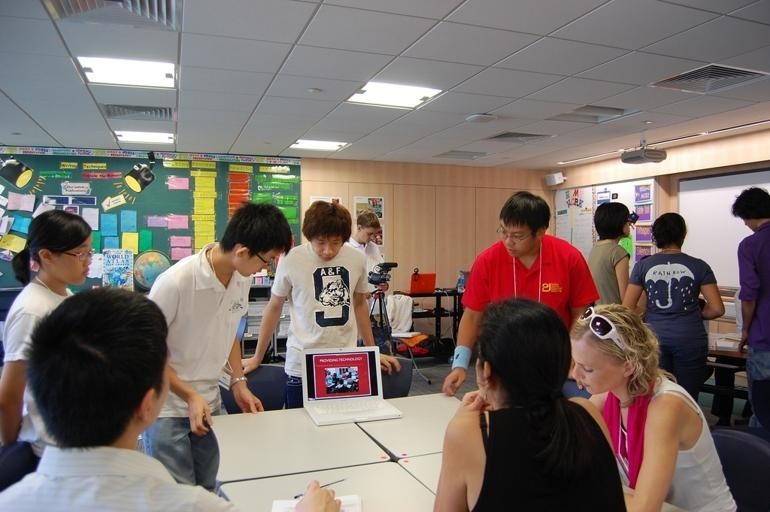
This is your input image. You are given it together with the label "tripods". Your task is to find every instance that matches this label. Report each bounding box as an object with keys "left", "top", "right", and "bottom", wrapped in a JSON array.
[{"left": 370, "top": 296, "right": 397, "bottom": 355}]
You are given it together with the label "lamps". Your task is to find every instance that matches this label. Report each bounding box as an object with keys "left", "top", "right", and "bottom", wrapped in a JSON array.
[
  {"left": 124, "top": 150, "right": 156, "bottom": 193},
  {"left": 0, "top": 159, "right": 33, "bottom": 189}
]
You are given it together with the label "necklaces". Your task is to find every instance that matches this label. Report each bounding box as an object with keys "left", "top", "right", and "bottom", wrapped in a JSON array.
[
  {"left": 660, "top": 246, "right": 681, "bottom": 255},
  {"left": 34, "top": 274, "right": 69, "bottom": 304},
  {"left": 513, "top": 237, "right": 544, "bottom": 306},
  {"left": 209, "top": 243, "right": 232, "bottom": 289}
]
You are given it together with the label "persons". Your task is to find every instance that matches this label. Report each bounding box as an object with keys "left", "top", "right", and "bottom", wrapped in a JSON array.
[
  {"left": 349, "top": 211, "right": 390, "bottom": 355},
  {"left": 571, "top": 301, "right": 740, "bottom": 512},
  {"left": 0, "top": 286, "right": 344, "bottom": 512},
  {"left": 0, "top": 210, "right": 96, "bottom": 473},
  {"left": 442, "top": 189, "right": 602, "bottom": 400},
  {"left": 326, "top": 369, "right": 360, "bottom": 392},
  {"left": 331, "top": 197, "right": 339, "bottom": 204},
  {"left": 242, "top": 199, "right": 401, "bottom": 409},
  {"left": 620, "top": 211, "right": 726, "bottom": 406},
  {"left": 140, "top": 202, "right": 294, "bottom": 494},
  {"left": 731, "top": 186, "right": 769, "bottom": 437},
  {"left": 587, "top": 201, "right": 631, "bottom": 306},
  {"left": 431, "top": 297, "right": 627, "bottom": 512}
]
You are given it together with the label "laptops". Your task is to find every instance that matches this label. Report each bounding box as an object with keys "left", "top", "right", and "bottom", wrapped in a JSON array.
[
  {"left": 400, "top": 272, "right": 437, "bottom": 295},
  {"left": 299, "top": 346, "right": 402, "bottom": 425}
]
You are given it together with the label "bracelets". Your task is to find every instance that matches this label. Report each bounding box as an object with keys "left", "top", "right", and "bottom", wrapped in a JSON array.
[
  {"left": 229, "top": 376, "right": 248, "bottom": 392},
  {"left": 451, "top": 345, "right": 472, "bottom": 371}
]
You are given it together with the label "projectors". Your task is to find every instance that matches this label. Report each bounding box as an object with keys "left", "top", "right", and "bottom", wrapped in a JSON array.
[{"left": 621, "top": 149, "right": 667, "bottom": 164}]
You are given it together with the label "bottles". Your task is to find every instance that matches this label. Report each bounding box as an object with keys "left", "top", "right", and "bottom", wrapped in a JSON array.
[{"left": 457, "top": 271, "right": 465, "bottom": 293}]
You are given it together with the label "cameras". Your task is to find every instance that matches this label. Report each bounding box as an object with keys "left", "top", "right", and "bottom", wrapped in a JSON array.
[{"left": 368, "top": 259, "right": 397, "bottom": 284}]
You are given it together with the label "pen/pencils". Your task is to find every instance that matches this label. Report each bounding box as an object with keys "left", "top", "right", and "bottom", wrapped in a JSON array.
[{"left": 294, "top": 478, "right": 345, "bottom": 499}]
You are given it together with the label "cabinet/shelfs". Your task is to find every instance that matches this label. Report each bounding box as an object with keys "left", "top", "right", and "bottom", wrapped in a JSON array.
[
  {"left": 242, "top": 285, "right": 274, "bottom": 362},
  {"left": 275, "top": 296, "right": 291, "bottom": 356},
  {"left": 392, "top": 288, "right": 458, "bottom": 367}
]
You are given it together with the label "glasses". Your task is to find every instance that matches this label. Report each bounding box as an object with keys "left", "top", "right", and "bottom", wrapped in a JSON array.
[
  {"left": 497, "top": 226, "right": 531, "bottom": 243},
  {"left": 62, "top": 249, "right": 92, "bottom": 261},
  {"left": 579, "top": 307, "right": 625, "bottom": 351},
  {"left": 254, "top": 250, "right": 275, "bottom": 265}
]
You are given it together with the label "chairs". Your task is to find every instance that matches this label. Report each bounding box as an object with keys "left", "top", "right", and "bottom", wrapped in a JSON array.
[
  {"left": 710, "top": 429, "right": 770, "bottom": 512},
  {"left": 0, "top": 441, "right": 37, "bottom": 493},
  {"left": 218, "top": 366, "right": 286, "bottom": 414},
  {"left": 381, "top": 358, "right": 412, "bottom": 399}
]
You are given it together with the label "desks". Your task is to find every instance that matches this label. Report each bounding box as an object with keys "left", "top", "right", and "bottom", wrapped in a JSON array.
[
  {"left": 398, "top": 453, "right": 686, "bottom": 512},
  {"left": 699, "top": 332, "right": 754, "bottom": 428},
  {"left": 699, "top": 295, "right": 737, "bottom": 334},
  {"left": 218, "top": 462, "right": 436, "bottom": 512},
  {"left": 207, "top": 408, "right": 390, "bottom": 490},
  {"left": 357, "top": 393, "right": 463, "bottom": 459}
]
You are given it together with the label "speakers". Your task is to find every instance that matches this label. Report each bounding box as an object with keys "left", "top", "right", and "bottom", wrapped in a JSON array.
[{"left": 545, "top": 172, "right": 564, "bottom": 186}]
